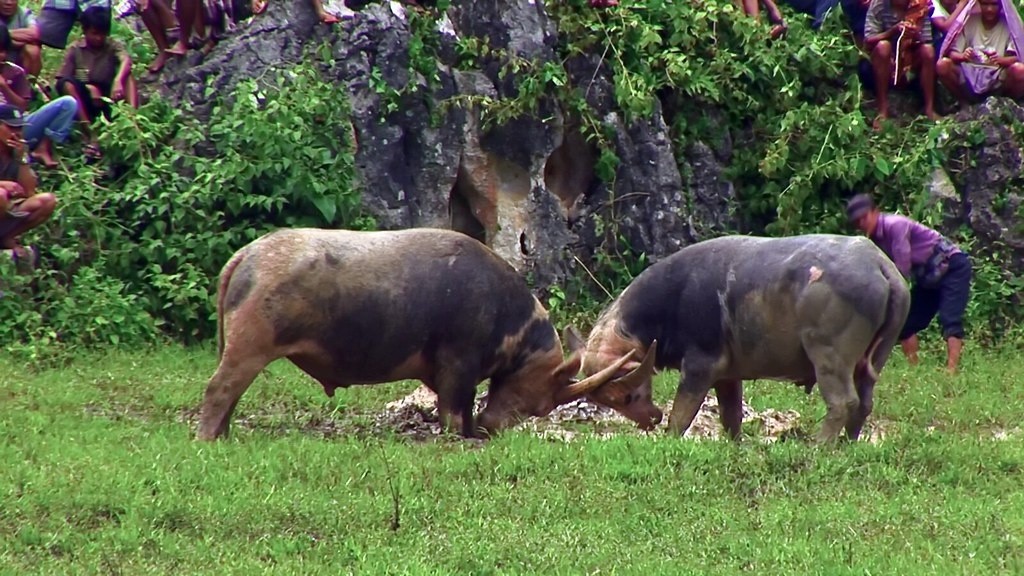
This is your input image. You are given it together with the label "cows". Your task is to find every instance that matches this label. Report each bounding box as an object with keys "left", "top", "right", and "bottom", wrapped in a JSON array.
[
  {"left": 193, "top": 222, "right": 640, "bottom": 444},
  {"left": 557, "top": 228, "right": 915, "bottom": 450}
]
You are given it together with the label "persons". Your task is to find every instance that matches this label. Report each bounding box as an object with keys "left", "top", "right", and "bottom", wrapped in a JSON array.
[
  {"left": 742, "top": 0, "right": 1024, "bottom": 132},
  {"left": 0, "top": 0, "right": 423, "bottom": 259},
  {"left": 844, "top": 194, "right": 972, "bottom": 375}
]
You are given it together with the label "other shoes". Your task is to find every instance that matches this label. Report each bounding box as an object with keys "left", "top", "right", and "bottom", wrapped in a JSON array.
[{"left": 82, "top": 143, "right": 101, "bottom": 160}]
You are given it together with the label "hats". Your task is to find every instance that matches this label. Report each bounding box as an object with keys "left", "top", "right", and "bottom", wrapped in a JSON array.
[
  {"left": 846, "top": 194, "right": 872, "bottom": 222},
  {"left": 0, "top": 104, "right": 33, "bottom": 128}
]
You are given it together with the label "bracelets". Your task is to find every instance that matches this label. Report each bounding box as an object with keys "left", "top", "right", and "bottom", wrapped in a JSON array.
[
  {"left": 16, "top": 152, "right": 31, "bottom": 165},
  {"left": 78, "top": 121, "right": 91, "bottom": 125}
]
